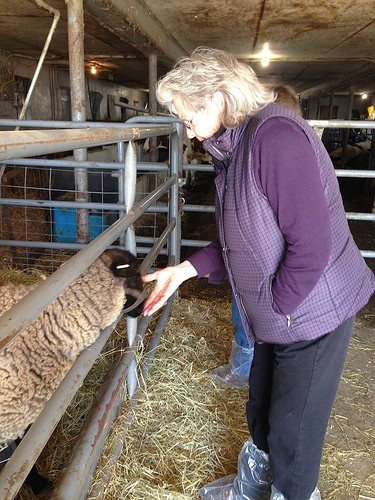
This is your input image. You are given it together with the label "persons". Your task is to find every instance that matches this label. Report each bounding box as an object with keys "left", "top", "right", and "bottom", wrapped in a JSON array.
[
  {"left": 140, "top": 45, "right": 375, "bottom": 499},
  {"left": 205, "top": 83, "right": 302, "bottom": 391}
]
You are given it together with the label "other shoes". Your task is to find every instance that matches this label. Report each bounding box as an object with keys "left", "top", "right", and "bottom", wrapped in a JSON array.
[{"left": 206, "top": 481, "right": 267, "bottom": 500}]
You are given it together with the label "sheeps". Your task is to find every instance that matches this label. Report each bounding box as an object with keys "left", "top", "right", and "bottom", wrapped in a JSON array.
[
  {"left": 0, "top": 248, "right": 144, "bottom": 494},
  {"left": 142, "top": 135, "right": 214, "bottom": 188}
]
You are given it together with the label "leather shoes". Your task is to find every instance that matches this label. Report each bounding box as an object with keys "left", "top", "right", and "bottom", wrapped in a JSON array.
[{"left": 207, "top": 366, "right": 247, "bottom": 387}]
